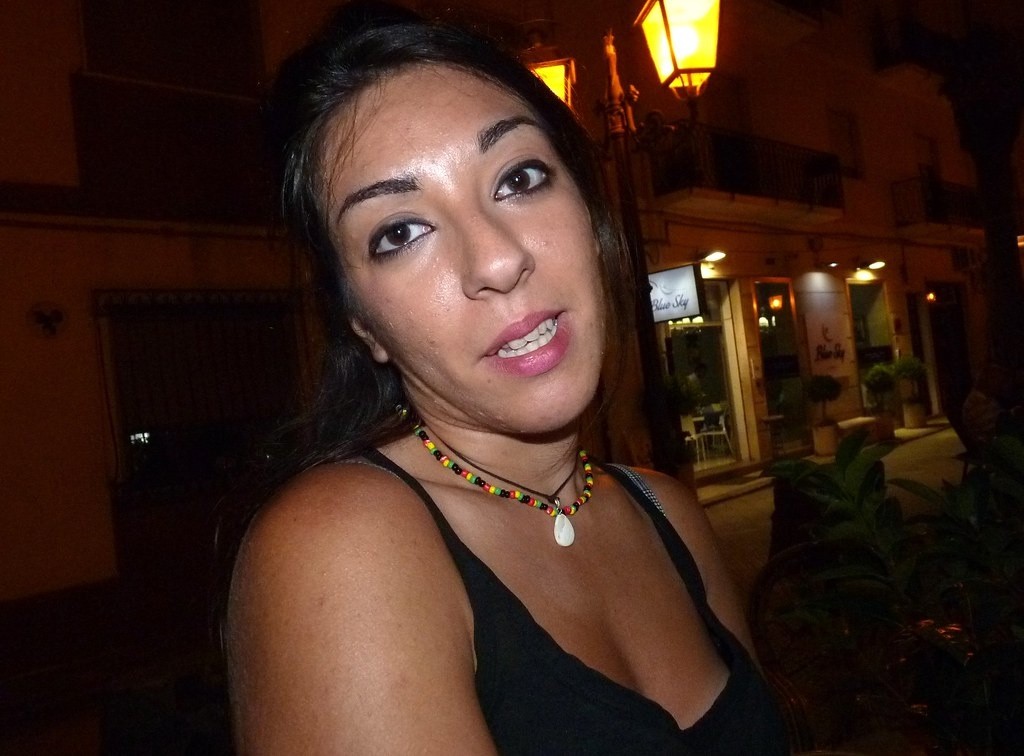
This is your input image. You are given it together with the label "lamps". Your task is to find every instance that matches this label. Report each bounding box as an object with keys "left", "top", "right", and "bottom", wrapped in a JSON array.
[
  {"left": 698, "top": 249, "right": 726, "bottom": 264},
  {"left": 860, "top": 251, "right": 886, "bottom": 270}
]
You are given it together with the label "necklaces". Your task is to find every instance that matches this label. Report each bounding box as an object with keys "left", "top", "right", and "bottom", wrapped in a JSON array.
[
  {"left": 402, "top": 411, "right": 593, "bottom": 515},
  {"left": 447, "top": 446, "right": 580, "bottom": 546}
]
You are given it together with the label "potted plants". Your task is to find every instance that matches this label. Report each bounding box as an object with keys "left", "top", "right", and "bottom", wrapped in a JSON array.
[
  {"left": 863, "top": 363, "right": 898, "bottom": 438},
  {"left": 805, "top": 375, "right": 838, "bottom": 458},
  {"left": 895, "top": 356, "right": 929, "bottom": 428}
]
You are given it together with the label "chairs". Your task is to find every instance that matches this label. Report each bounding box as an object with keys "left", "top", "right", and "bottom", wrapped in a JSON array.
[
  {"left": 679, "top": 414, "right": 706, "bottom": 463},
  {"left": 702, "top": 406, "right": 733, "bottom": 461}
]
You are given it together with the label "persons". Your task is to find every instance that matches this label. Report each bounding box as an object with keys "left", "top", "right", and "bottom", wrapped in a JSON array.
[{"left": 226, "top": 24, "right": 805, "bottom": 756}]
